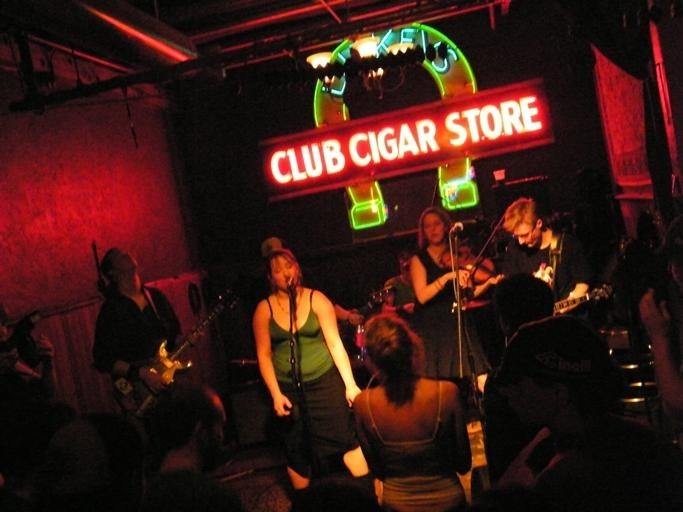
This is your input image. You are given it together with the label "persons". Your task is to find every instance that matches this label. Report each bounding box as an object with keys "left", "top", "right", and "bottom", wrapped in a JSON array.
[
  {"left": 498, "top": 195, "right": 592, "bottom": 321},
  {"left": 407, "top": 206, "right": 505, "bottom": 414},
  {"left": 662, "top": 214, "right": 682, "bottom": 293},
  {"left": 88, "top": 247, "right": 227, "bottom": 468},
  {"left": 380, "top": 244, "right": 423, "bottom": 328},
  {"left": 250, "top": 235, "right": 370, "bottom": 491},
  {"left": 0, "top": 268, "right": 683, "bottom": 512}
]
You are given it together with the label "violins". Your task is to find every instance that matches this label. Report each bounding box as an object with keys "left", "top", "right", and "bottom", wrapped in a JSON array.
[{"left": 439, "top": 239, "right": 499, "bottom": 283}]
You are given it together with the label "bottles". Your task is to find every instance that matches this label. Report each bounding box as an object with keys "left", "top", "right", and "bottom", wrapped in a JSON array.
[{"left": 352, "top": 321, "right": 366, "bottom": 360}]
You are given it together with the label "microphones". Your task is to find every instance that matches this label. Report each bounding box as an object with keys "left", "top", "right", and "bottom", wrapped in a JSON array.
[
  {"left": 454, "top": 216, "right": 485, "bottom": 233},
  {"left": 285, "top": 276, "right": 297, "bottom": 300}
]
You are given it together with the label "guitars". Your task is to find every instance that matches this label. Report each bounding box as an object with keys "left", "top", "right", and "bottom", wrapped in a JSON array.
[
  {"left": 553, "top": 284, "right": 615, "bottom": 312},
  {"left": 112, "top": 288, "right": 240, "bottom": 419}
]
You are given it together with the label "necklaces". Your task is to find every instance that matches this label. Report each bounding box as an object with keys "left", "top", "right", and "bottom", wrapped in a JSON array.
[{"left": 276, "top": 287, "right": 301, "bottom": 314}]
